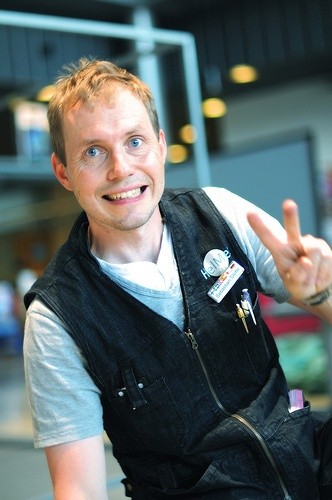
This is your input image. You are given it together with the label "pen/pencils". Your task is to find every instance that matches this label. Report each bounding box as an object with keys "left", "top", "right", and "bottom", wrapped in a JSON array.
[
  {"left": 240, "top": 288, "right": 258, "bottom": 325},
  {"left": 236, "top": 304, "right": 250, "bottom": 333}
]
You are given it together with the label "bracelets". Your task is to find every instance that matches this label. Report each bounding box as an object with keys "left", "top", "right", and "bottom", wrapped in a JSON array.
[{"left": 300, "top": 285, "right": 331, "bottom": 307}]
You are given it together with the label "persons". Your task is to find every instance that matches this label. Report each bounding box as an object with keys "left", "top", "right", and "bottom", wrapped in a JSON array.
[{"left": 20, "top": 57, "right": 332, "bottom": 500}]
